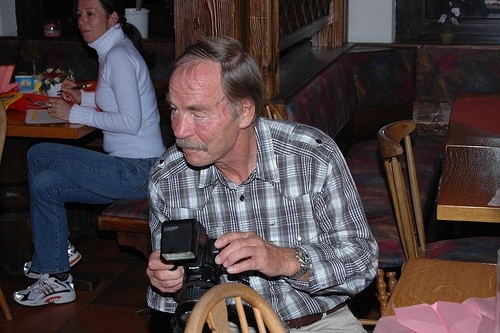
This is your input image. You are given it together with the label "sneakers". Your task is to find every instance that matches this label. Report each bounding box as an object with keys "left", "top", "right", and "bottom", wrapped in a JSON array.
[
  {"left": 13, "top": 273, "right": 76, "bottom": 306},
  {"left": 24, "top": 240, "right": 82, "bottom": 279}
]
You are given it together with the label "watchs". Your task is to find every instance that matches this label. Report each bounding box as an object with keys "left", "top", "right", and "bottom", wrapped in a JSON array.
[{"left": 289, "top": 247, "right": 311, "bottom": 279}]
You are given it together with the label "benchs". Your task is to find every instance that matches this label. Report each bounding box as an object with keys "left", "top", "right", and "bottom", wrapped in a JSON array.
[
  {"left": 0, "top": 34, "right": 175, "bottom": 260},
  {"left": 285, "top": 45, "right": 500, "bottom": 326}
]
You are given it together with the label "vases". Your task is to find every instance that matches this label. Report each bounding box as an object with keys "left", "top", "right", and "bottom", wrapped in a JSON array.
[{"left": 46, "top": 82, "right": 62, "bottom": 97}]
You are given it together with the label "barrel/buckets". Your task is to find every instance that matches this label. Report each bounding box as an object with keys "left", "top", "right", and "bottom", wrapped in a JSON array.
[{"left": 125, "top": 8, "right": 150, "bottom": 39}]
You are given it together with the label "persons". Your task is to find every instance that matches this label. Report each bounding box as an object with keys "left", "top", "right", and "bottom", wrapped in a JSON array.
[
  {"left": 12, "top": 0, "right": 166, "bottom": 307},
  {"left": 147, "top": 36, "right": 379, "bottom": 333}
]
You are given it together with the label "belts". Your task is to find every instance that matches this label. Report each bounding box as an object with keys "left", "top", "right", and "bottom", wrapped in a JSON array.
[{"left": 227, "top": 301, "right": 348, "bottom": 330}]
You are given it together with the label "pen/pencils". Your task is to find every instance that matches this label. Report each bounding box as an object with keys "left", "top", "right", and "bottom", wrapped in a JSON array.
[{"left": 57, "top": 84, "right": 92, "bottom": 92}]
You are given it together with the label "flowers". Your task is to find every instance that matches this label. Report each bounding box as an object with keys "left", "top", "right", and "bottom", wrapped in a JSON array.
[{"left": 37, "top": 64, "right": 68, "bottom": 93}]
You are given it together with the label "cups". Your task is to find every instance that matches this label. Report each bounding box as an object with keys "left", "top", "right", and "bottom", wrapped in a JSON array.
[{"left": 43, "top": 21, "right": 61, "bottom": 37}]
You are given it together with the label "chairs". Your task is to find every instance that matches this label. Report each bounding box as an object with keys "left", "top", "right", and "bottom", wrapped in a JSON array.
[
  {"left": 184, "top": 283, "right": 289, "bottom": 333},
  {"left": 376, "top": 120, "right": 500, "bottom": 273}
]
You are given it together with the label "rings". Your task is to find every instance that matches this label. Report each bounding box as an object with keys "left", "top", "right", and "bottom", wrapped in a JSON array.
[{"left": 49, "top": 102, "right": 53, "bottom": 107}]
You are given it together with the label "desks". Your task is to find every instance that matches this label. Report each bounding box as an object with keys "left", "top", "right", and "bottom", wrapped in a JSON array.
[
  {"left": 5, "top": 79, "right": 108, "bottom": 293},
  {"left": 436, "top": 147, "right": 500, "bottom": 223},
  {"left": 372, "top": 258, "right": 500, "bottom": 333},
  {"left": 446, "top": 90, "right": 500, "bottom": 147}
]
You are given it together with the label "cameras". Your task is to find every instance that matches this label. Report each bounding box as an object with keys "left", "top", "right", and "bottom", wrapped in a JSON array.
[{"left": 159, "top": 205, "right": 239, "bottom": 332}]
